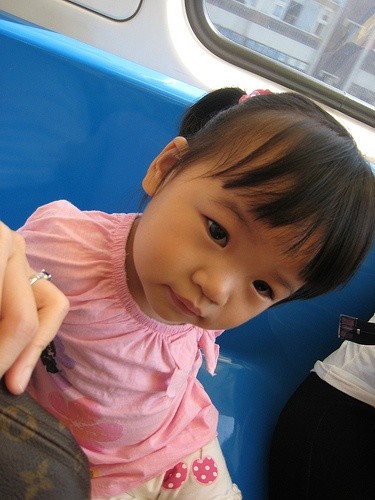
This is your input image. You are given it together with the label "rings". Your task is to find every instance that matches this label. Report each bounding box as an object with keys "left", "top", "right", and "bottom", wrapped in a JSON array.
[{"left": 28, "top": 269, "right": 52, "bottom": 287}]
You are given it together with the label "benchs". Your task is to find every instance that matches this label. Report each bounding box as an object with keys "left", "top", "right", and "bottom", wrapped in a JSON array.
[{"left": 0, "top": 10, "right": 375, "bottom": 500}]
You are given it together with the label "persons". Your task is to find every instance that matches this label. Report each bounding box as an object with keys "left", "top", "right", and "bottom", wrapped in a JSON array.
[
  {"left": 267, "top": 312, "right": 375, "bottom": 500},
  {"left": 0, "top": 220, "right": 70, "bottom": 395},
  {"left": 15, "top": 87, "right": 375, "bottom": 500}
]
